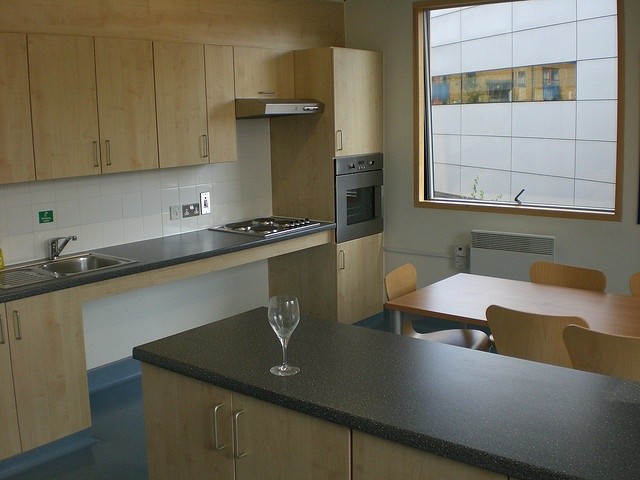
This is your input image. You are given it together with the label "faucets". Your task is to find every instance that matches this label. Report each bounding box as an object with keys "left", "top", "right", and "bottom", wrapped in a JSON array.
[{"left": 54, "top": 235, "right": 77, "bottom": 261}]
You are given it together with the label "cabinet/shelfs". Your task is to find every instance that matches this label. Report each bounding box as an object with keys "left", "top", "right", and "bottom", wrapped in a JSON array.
[
  {"left": 351, "top": 427, "right": 513, "bottom": 480},
  {"left": 139, "top": 359, "right": 351, "bottom": 479},
  {"left": 0, "top": 278, "right": 93, "bottom": 463},
  {"left": 26, "top": 30, "right": 160, "bottom": 181},
  {"left": 267, "top": 230, "right": 385, "bottom": 328},
  {"left": 233, "top": 48, "right": 297, "bottom": 101},
  {"left": 0, "top": 32, "right": 36, "bottom": 183},
  {"left": 269, "top": 48, "right": 386, "bottom": 158},
  {"left": 152, "top": 37, "right": 237, "bottom": 168}
]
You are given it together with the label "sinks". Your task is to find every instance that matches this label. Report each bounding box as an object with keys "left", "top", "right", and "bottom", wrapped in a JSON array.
[{"left": 31, "top": 252, "right": 139, "bottom": 278}]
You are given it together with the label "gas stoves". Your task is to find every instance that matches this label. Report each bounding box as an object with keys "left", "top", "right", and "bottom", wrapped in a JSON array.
[{"left": 207, "top": 216, "right": 321, "bottom": 238}]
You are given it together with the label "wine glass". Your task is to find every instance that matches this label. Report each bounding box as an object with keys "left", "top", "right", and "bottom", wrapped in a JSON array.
[{"left": 267, "top": 295, "right": 301, "bottom": 377}]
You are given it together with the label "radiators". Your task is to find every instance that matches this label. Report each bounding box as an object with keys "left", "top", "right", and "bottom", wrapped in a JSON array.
[{"left": 469, "top": 228, "right": 555, "bottom": 283}]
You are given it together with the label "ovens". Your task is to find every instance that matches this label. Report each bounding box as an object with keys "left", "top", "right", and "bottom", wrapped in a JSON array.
[{"left": 334, "top": 153, "right": 383, "bottom": 244}]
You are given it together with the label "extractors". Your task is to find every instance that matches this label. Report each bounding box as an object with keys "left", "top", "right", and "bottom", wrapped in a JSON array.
[{"left": 235, "top": 98, "right": 325, "bottom": 119}]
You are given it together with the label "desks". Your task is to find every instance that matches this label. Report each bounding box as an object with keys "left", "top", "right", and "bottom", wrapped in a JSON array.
[{"left": 383, "top": 271, "right": 639, "bottom": 337}]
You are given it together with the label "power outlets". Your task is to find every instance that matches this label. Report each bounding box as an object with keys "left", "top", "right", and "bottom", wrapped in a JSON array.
[
  {"left": 455, "top": 243, "right": 467, "bottom": 270},
  {"left": 183, "top": 204, "right": 200, "bottom": 218},
  {"left": 200, "top": 192, "right": 211, "bottom": 214},
  {"left": 170, "top": 205, "right": 181, "bottom": 219}
]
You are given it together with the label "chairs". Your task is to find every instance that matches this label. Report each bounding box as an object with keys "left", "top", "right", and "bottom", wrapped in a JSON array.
[
  {"left": 484, "top": 304, "right": 591, "bottom": 369},
  {"left": 629, "top": 272, "right": 640, "bottom": 297},
  {"left": 563, "top": 323, "right": 640, "bottom": 383},
  {"left": 380, "top": 262, "right": 491, "bottom": 351},
  {"left": 528, "top": 260, "right": 607, "bottom": 294}
]
